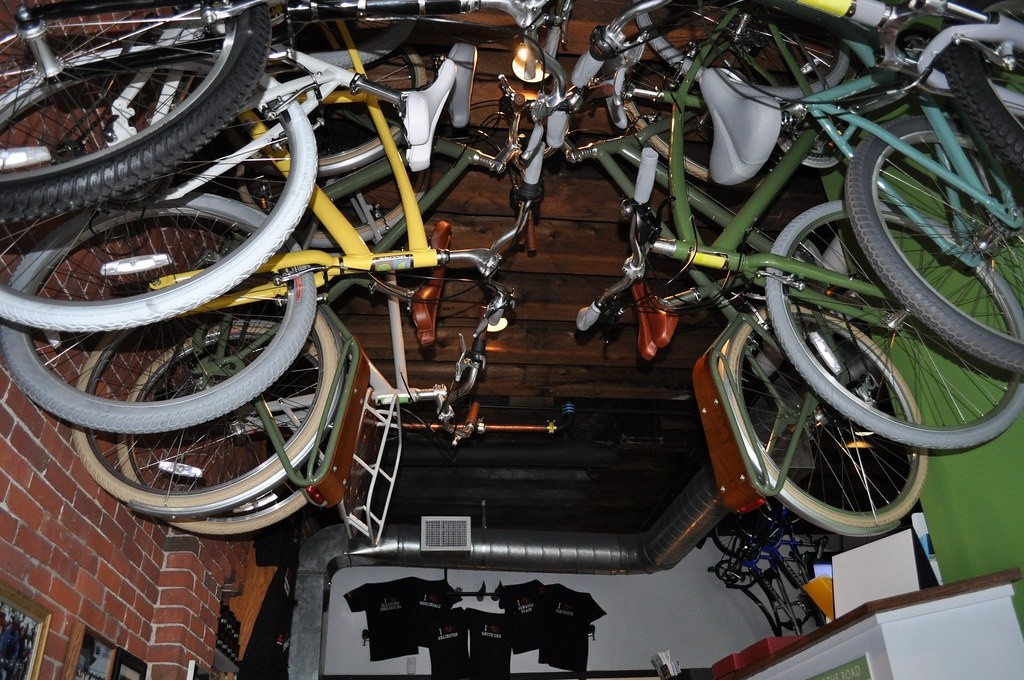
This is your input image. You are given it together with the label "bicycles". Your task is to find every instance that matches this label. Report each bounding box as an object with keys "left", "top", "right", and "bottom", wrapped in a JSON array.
[
  {"left": 0, "top": 1, "right": 559, "bottom": 547},
  {"left": 567, "top": 0, "right": 1024, "bottom": 536}
]
